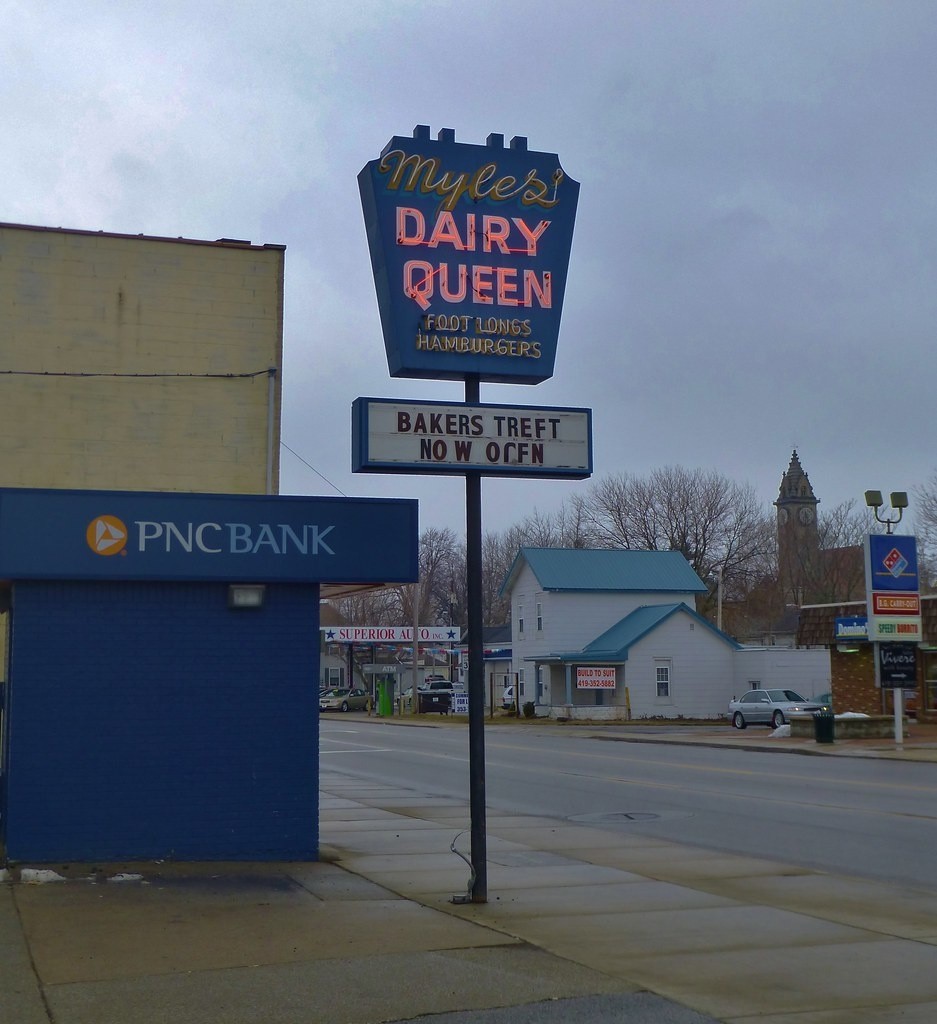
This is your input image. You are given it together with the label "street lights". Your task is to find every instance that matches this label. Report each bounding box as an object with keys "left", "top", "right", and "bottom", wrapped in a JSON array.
[{"left": 863, "top": 490, "right": 909, "bottom": 743}]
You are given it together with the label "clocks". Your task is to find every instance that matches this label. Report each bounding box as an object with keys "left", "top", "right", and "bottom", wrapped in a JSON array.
[
  {"left": 777, "top": 508, "right": 789, "bottom": 526},
  {"left": 800, "top": 507, "right": 814, "bottom": 525}
]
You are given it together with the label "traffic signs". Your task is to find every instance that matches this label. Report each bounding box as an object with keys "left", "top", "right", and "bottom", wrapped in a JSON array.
[{"left": 874, "top": 641, "right": 919, "bottom": 689}]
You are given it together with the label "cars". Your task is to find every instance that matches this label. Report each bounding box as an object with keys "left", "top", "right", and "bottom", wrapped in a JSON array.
[
  {"left": 319, "top": 687, "right": 375, "bottom": 712},
  {"left": 728, "top": 689, "right": 832, "bottom": 730},
  {"left": 501, "top": 685, "right": 512, "bottom": 709},
  {"left": 397, "top": 680, "right": 464, "bottom": 708}
]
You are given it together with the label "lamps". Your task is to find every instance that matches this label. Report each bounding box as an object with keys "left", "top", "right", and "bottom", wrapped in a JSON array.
[{"left": 229, "top": 583, "right": 268, "bottom": 612}]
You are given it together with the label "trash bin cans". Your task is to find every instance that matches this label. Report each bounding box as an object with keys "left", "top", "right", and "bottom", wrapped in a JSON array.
[
  {"left": 418, "top": 691, "right": 449, "bottom": 715},
  {"left": 811, "top": 712, "right": 834, "bottom": 743}
]
